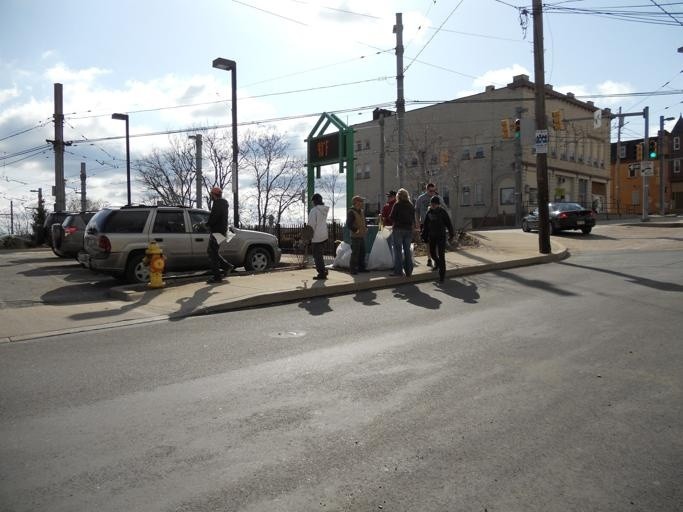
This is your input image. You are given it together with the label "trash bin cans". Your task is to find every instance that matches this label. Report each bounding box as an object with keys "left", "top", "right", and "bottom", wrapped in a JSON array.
[{"left": 364, "top": 217, "right": 379, "bottom": 254}]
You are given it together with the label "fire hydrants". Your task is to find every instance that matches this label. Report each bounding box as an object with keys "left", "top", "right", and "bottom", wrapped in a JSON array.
[{"left": 142, "top": 238, "right": 166, "bottom": 290}]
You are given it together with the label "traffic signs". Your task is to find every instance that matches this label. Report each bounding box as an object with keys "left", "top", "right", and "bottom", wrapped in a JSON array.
[{"left": 641, "top": 161, "right": 654, "bottom": 176}]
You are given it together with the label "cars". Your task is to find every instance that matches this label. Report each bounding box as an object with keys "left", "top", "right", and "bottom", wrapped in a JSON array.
[{"left": 520, "top": 201, "right": 598, "bottom": 238}]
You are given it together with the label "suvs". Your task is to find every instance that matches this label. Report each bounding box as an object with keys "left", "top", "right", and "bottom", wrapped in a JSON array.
[{"left": 31, "top": 200, "right": 283, "bottom": 282}]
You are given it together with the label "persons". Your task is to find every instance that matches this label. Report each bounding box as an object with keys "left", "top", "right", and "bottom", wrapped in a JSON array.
[
  {"left": 307, "top": 193, "right": 330, "bottom": 280},
  {"left": 205, "top": 187, "right": 234, "bottom": 284},
  {"left": 381, "top": 190, "right": 396, "bottom": 226},
  {"left": 345, "top": 195, "right": 370, "bottom": 275},
  {"left": 415, "top": 182, "right": 447, "bottom": 267},
  {"left": 422, "top": 195, "right": 454, "bottom": 284},
  {"left": 389, "top": 187, "right": 415, "bottom": 276}
]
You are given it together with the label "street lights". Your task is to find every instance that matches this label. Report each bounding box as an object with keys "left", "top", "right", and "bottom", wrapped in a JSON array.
[
  {"left": 211, "top": 56, "right": 241, "bottom": 230},
  {"left": 187, "top": 134, "right": 203, "bottom": 210},
  {"left": 110, "top": 112, "right": 132, "bottom": 210},
  {"left": 658, "top": 115, "right": 675, "bottom": 216},
  {"left": 367, "top": 104, "right": 398, "bottom": 224}
]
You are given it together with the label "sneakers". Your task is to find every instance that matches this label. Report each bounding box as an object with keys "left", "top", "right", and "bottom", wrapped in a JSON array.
[
  {"left": 427, "top": 258, "right": 447, "bottom": 283},
  {"left": 350, "top": 268, "right": 370, "bottom": 275},
  {"left": 312, "top": 268, "right": 329, "bottom": 280},
  {"left": 388, "top": 271, "right": 412, "bottom": 278},
  {"left": 206, "top": 265, "right": 235, "bottom": 283}
]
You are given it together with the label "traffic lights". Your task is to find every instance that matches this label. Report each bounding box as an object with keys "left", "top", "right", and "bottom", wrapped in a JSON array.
[
  {"left": 500, "top": 119, "right": 510, "bottom": 140},
  {"left": 639, "top": 142, "right": 644, "bottom": 159},
  {"left": 512, "top": 117, "right": 520, "bottom": 141},
  {"left": 552, "top": 110, "right": 561, "bottom": 132},
  {"left": 636, "top": 144, "right": 642, "bottom": 161},
  {"left": 648, "top": 141, "right": 657, "bottom": 162}
]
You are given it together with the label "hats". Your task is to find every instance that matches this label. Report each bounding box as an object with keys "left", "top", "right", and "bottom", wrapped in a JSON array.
[
  {"left": 209, "top": 187, "right": 223, "bottom": 196},
  {"left": 309, "top": 189, "right": 440, "bottom": 205}
]
